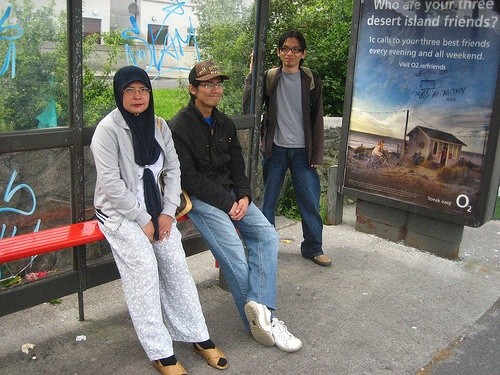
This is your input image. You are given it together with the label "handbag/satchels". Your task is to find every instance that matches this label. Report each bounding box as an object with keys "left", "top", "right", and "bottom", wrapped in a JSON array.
[{"left": 159, "top": 171, "right": 192, "bottom": 219}]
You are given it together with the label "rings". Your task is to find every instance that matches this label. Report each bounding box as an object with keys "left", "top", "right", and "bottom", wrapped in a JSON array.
[{"left": 164, "top": 231, "right": 166, "bottom": 234}]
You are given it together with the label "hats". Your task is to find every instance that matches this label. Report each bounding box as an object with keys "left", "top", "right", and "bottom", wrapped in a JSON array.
[{"left": 189, "top": 60, "right": 229, "bottom": 84}]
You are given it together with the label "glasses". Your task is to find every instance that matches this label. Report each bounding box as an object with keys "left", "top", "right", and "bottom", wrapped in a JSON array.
[
  {"left": 280, "top": 46, "right": 304, "bottom": 53},
  {"left": 196, "top": 82, "right": 225, "bottom": 89},
  {"left": 123, "top": 87, "right": 151, "bottom": 96}
]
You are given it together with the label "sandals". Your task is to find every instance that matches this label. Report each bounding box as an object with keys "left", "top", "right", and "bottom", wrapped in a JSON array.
[
  {"left": 152, "top": 356, "right": 188, "bottom": 375},
  {"left": 193, "top": 339, "right": 228, "bottom": 369}
]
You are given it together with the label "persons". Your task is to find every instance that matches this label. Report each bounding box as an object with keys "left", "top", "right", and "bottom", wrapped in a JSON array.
[
  {"left": 242, "top": 31, "right": 333, "bottom": 268},
  {"left": 169, "top": 60, "right": 304, "bottom": 352},
  {"left": 90, "top": 65, "right": 229, "bottom": 375},
  {"left": 371, "top": 141, "right": 387, "bottom": 163}
]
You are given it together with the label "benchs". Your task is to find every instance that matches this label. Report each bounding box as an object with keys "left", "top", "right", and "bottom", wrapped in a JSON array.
[{"left": 0, "top": 212, "right": 238, "bottom": 321}]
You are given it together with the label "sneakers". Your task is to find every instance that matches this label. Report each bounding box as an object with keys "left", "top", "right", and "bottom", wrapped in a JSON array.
[
  {"left": 311, "top": 253, "right": 332, "bottom": 265},
  {"left": 271, "top": 318, "right": 302, "bottom": 352},
  {"left": 244, "top": 301, "right": 275, "bottom": 347}
]
